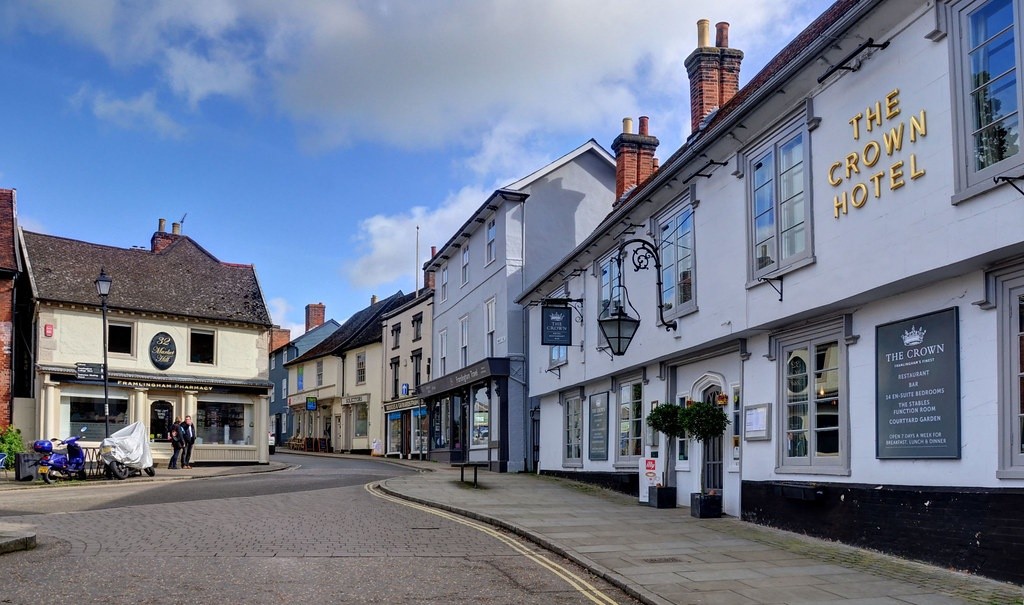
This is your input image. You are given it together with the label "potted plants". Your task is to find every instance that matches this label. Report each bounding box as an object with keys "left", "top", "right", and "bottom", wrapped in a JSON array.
[
  {"left": 646, "top": 400, "right": 683, "bottom": 509},
  {"left": 677, "top": 401, "right": 733, "bottom": 518}
]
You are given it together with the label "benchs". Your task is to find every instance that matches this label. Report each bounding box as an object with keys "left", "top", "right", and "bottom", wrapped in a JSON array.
[{"left": 451, "top": 463, "right": 489, "bottom": 488}]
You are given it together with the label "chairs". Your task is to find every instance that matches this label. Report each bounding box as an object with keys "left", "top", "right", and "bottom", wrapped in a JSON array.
[{"left": 0, "top": 453, "right": 10, "bottom": 483}]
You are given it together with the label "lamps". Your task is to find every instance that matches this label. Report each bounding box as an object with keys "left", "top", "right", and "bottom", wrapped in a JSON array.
[{"left": 596, "top": 238, "right": 677, "bottom": 356}]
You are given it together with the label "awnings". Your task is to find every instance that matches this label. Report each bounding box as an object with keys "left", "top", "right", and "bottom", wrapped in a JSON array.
[{"left": 106, "top": 368, "right": 274, "bottom": 395}]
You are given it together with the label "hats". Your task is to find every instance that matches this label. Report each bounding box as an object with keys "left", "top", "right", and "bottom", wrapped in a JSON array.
[{"left": 175, "top": 416, "right": 181, "bottom": 421}]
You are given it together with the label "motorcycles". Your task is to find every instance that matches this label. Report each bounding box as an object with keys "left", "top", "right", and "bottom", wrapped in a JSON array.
[
  {"left": 34, "top": 426, "right": 88, "bottom": 484},
  {"left": 99, "top": 420, "right": 156, "bottom": 480}
]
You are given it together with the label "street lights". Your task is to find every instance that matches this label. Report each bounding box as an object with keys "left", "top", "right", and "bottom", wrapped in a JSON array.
[{"left": 93, "top": 266, "right": 113, "bottom": 480}]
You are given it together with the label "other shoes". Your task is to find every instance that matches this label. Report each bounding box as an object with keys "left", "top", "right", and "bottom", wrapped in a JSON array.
[
  {"left": 187, "top": 466, "right": 191, "bottom": 469},
  {"left": 183, "top": 466, "right": 187, "bottom": 469},
  {"left": 174, "top": 466, "right": 178, "bottom": 469},
  {"left": 168, "top": 466, "right": 172, "bottom": 469}
]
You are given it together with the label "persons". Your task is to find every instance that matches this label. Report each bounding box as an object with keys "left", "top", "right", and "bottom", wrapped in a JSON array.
[
  {"left": 787, "top": 432, "right": 797, "bottom": 457},
  {"left": 168, "top": 415, "right": 186, "bottom": 469},
  {"left": 180, "top": 415, "right": 195, "bottom": 469}
]
